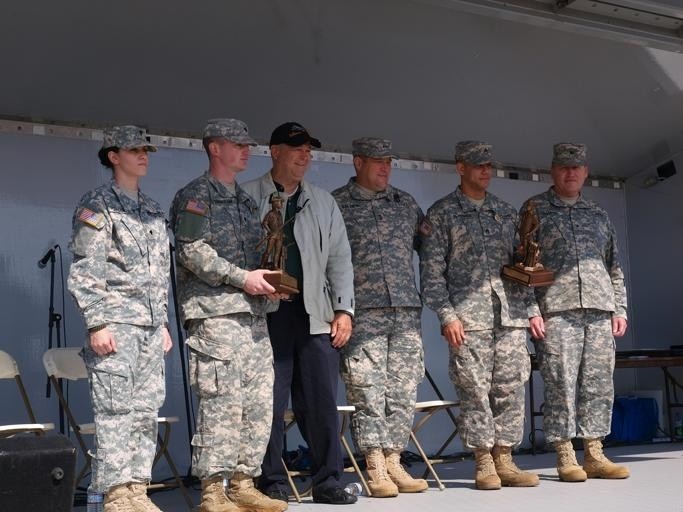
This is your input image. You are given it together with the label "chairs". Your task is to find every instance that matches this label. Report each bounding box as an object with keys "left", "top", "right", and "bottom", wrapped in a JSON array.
[
  {"left": 0, "top": 351, "right": 56, "bottom": 436},
  {"left": 42, "top": 347, "right": 196, "bottom": 510}
]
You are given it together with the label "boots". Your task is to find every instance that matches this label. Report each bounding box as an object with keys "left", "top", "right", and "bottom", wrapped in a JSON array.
[
  {"left": 554, "top": 436, "right": 587, "bottom": 482},
  {"left": 362, "top": 442, "right": 403, "bottom": 498},
  {"left": 130, "top": 481, "right": 161, "bottom": 512},
  {"left": 99, "top": 483, "right": 137, "bottom": 512},
  {"left": 474, "top": 446, "right": 502, "bottom": 490},
  {"left": 581, "top": 437, "right": 631, "bottom": 480},
  {"left": 380, "top": 446, "right": 429, "bottom": 493},
  {"left": 199, "top": 471, "right": 249, "bottom": 512},
  {"left": 227, "top": 470, "right": 289, "bottom": 512},
  {"left": 492, "top": 444, "right": 541, "bottom": 489}
]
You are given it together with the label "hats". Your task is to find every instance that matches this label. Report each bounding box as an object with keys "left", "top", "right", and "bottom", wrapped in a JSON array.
[
  {"left": 269, "top": 121, "right": 324, "bottom": 148},
  {"left": 552, "top": 142, "right": 589, "bottom": 168},
  {"left": 352, "top": 136, "right": 401, "bottom": 160},
  {"left": 455, "top": 140, "right": 505, "bottom": 166},
  {"left": 200, "top": 117, "right": 258, "bottom": 148},
  {"left": 98, "top": 124, "right": 158, "bottom": 152}
]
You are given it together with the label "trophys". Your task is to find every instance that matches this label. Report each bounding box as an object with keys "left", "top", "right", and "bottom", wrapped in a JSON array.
[
  {"left": 255, "top": 197, "right": 300, "bottom": 294},
  {"left": 501, "top": 201, "right": 553, "bottom": 286}
]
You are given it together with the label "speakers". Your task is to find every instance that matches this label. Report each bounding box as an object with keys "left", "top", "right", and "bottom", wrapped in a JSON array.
[{"left": 1, "top": 435, "right": 76, "bottom": 511}]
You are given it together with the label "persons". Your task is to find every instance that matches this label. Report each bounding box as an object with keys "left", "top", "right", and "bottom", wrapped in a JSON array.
[
  {"left": 518, "top": 142, "right": 629, "bottom": 482},
  {"left": 329, "top": 135, "right": 430, "bottom": 500},
  {"left": 68, "top": 125, "right": 170, "bottom": 512},
  {"left": 172, "top": 118, "right": 289, "bottom": 512},
  {"left": 418, "top": 140, "right": 545, "bottom": 491},
  {"left": 236, "top": 120, "right": 356, "bottom": 507}
]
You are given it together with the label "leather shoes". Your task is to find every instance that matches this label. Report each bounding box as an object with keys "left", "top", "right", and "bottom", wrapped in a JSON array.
[
  {"left": 261, "top": 476, "right": 288, "bottom": 500},
  {"left": 308, "top": 481, "right": 357, "bottom": 504}
]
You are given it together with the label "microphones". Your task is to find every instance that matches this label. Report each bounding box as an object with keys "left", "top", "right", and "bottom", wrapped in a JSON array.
[{"left": 38, "top": 244, "right": 60, "bottom": 269}]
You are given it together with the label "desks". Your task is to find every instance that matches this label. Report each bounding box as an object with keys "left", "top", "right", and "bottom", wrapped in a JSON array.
[{"left": 531, "top": 347, "right": 683, "bottom": 456}]
